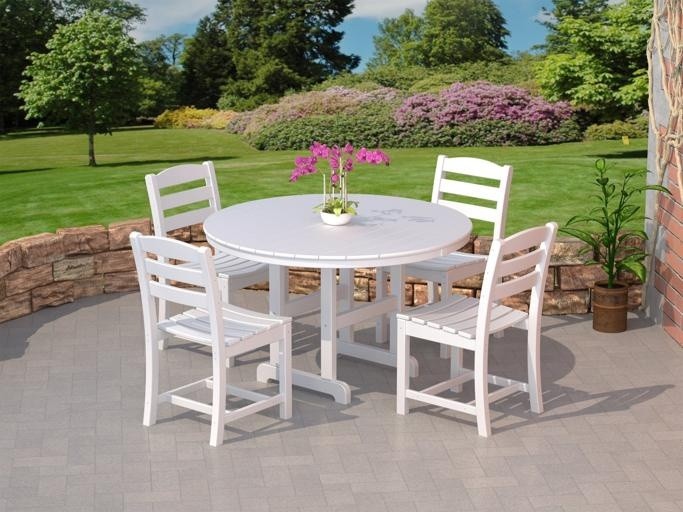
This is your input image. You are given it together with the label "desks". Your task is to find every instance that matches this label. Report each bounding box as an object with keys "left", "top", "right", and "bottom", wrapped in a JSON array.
[{"left": 202, "top": 193, "right": 472, "bottom": 405}]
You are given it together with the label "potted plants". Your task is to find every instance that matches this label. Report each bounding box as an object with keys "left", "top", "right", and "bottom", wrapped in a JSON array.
[
  {"left": 288, "top": 141, "right": 390, "bottom": 226},
  {"left": 558, "top": 159, "right": 672, "bottom": 333}
]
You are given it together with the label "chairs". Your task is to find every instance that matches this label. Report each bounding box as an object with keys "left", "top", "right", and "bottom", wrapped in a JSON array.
[
  {"left": 389, "top": 222, "right": 558, "bottom": 438},
  {"left": 145, "top": 163, "right": 288, "bottom": 369},
  {"left": 129, "top": 230, "right": 292, "bottom": 446},
  {"left": 377, "top": 157, "right": 512, "bottom": 357}
]
